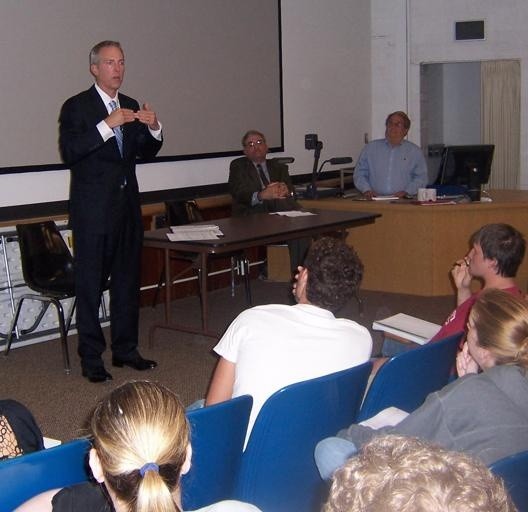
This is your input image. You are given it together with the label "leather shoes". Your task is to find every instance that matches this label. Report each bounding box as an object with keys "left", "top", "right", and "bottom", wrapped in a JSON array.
[
  {"left": 82, "top": 363, "right": 112, "bottom": 383},
  {"left": 111, "top": 350, "right": 158, "bottom": 371}
]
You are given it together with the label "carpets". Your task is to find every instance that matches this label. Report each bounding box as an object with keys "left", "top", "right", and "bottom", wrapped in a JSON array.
[{"left": 0, "top": 280, "right": 474, "bottom": 447}]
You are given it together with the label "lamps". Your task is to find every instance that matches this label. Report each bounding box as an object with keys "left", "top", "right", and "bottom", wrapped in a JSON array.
[{"left": 305, "top": 155, "right": 353, "bottom": 200}]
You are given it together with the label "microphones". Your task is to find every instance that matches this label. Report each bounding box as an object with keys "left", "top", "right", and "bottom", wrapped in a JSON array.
[{"left": 306, "top": 157, "right": 352, "bottom": 189}]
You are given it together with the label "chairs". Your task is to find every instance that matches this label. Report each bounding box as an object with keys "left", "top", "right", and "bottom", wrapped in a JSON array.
[
  {"left": 137, "top": 202, "right": 208, "bottom": 310},
  {"left": 360, "top": 332, "right": 464, "bottom": 424},
  {"left": 181, "top": 394, "right": 253, "bottom": 510},
  {"left": 243, "top": 362, "right": 372, "bottom": 512},
  {"left": 186, "top": 192, "right": 271, "bottom": 298},
  {"left": 0, "top": 433, "right": 104, "bottom": 512},
  {"left": 30, "top": 220, "right": 108, "bottom": 377},
  {"left": 482, "top": 448, "right": 526, "bottom": 512}
]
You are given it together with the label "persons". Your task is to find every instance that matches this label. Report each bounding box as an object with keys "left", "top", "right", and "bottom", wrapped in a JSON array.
[
  {"left": 318, "top": 433, "right": 520, "bottom": 512},
  {"left": 186, "top": 237, "right": 373, "bottom": 453},
  {"left": 353, "top": 111, "right": 429, "bottom": 199},
  {"left": 57, "top": 41, "right": 164, "bottom": 382},
  {"left": 0, "top": 399, "right": 46, "bottom": 459},
  {"left": 230, "top": 130, "right": 297, "bottom": 216},
  {"left": 381, "top": 223, "right": 526, "bottom": 358},
  {"left": 316, "top": 289, "right": 528, "bottom": 486},
  {"left": 11, "top": 370, "right": 193, "bottom": 512}
]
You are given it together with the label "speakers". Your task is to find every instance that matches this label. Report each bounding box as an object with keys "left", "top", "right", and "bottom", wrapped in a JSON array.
[{"left": 456, "top": 20, "right": 484, "bottom": 40}]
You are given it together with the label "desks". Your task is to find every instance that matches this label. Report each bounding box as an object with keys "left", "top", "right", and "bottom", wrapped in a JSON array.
[
  {"left": 294, "top": 188, "right": 528, "bottom": 296},
  {"left": 141, "top": 206, "right": 382, "bottom": 340}
]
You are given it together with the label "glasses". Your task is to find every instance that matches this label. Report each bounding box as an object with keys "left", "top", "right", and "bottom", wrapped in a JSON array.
[
  {"left": 386, "top": 121, "right": 404, "bottom": 128},
  {"left": 248, "top": 139, "right": 262, "bottom": 146}
]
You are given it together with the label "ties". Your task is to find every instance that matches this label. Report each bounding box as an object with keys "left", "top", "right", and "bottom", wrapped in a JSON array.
[
  {"left": 254, "top": 161, "right": 270, "bottom": 187},
  {"left": 109, "top": 100, "right": 124, "bottom": 158}
]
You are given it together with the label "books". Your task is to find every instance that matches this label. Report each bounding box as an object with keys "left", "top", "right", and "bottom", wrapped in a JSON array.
[
  {"left": 166, "top": 225, "right": 225, "bottom": 242},
  {"left": 268, "top": 210, "right": 317, "bottom": 218},
  {"left": 372, "top": 195, "right": 399, "bottom": 201},
  {"left": 372, "top": 312, "right": 444, "bottom": 345}
]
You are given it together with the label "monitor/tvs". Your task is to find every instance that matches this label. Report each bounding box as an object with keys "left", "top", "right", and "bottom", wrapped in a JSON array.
[{"left": 438, "top": 145, "right": 495, "bottom": 203}]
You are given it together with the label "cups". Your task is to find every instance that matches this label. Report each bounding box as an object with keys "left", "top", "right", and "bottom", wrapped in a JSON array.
[
  {"left": 416, "top": 188, "right": 425, "bottom": 201},
  {"left": 422, "top": 188, "right": 436, "bottom": 202}
]
